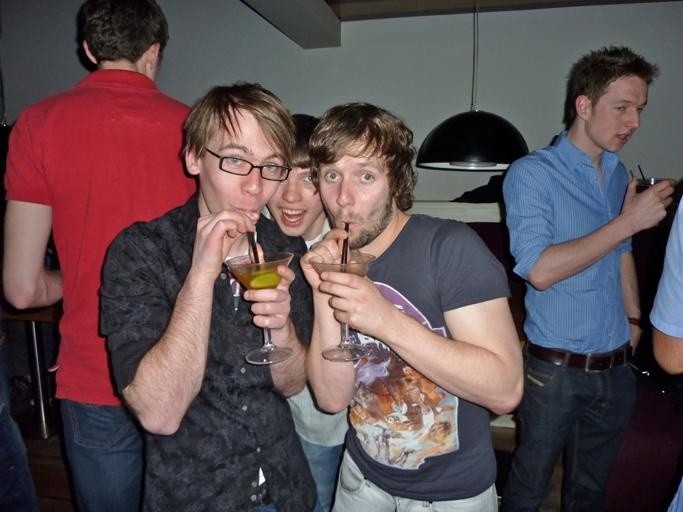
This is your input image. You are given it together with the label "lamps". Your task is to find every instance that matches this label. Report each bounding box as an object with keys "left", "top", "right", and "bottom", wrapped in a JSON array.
[{"left": 415, "top": 0, "right": 530, "bottom": 172}]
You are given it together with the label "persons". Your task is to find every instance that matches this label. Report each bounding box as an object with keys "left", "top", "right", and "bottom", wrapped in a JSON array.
[
  {"left": 267, "top": 113, "right": 350, "bottom": 512},
  {"left": 649, "top": 196, "right": 683, "bottom": 511},
  {"left": 503, "top": 47, "right": 675, "bottom": 512},
  {"left": 98, "top": 81, "right": 319, "bottom": 511},
  {"left": 300, "top": 104, "right": 524, "bottom": 512},
  {"left": 4, "top": 2, "right": 193, "bottom": 511},
  {"left": 1, "top": 320, "right": 41, "bottom": 512}
]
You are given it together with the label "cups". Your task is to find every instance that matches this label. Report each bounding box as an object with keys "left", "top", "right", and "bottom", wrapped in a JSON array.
[{"left": 635, "top": 178, "right": 674, "bottom": 196}]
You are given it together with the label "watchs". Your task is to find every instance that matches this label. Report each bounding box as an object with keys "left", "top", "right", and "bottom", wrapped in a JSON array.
[{"left": 629, "top": 317, "right": 646, "bottom": 329}]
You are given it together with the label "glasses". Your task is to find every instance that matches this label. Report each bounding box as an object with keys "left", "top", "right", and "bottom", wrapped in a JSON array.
[{"left": 201, "top": 146, "right": 293, "bottom": 183}]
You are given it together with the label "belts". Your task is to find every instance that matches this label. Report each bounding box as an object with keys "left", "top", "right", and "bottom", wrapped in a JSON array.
[{"left": 520, "top": 338, "right": 634, "bottom": 376}]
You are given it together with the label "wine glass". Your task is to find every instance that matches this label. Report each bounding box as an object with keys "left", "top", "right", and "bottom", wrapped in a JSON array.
[
  {"left": 223, "top": 252, "right": 295, "bottom": 365},
  {"left": 308, "top": 252, "right": 376, "bottom": 362}
]
